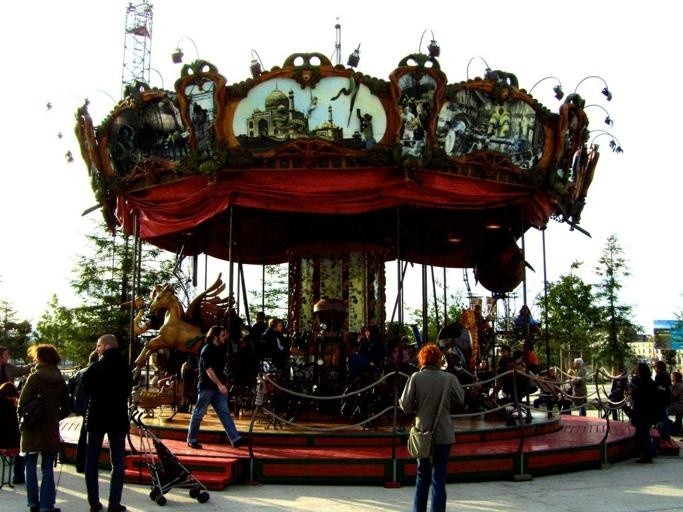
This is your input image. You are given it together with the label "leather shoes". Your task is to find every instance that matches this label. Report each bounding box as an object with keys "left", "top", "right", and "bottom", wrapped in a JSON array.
[
  {"left": 231, "top": 438, "right": 246, "bottom": 448},
  {"left": 189, "top": 442, "right": 203, "bottom": 449}
]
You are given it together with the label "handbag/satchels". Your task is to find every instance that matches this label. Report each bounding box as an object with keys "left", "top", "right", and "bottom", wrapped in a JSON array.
[
  {"left": 406, "top": 427, "right": 432, "bottom": 459},
  {"left": 18, "top": 393, "right": 45, "bottom": 432}
]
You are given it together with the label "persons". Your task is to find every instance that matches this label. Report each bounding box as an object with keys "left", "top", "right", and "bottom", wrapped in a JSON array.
[
  {"left": 0, "top": 381, "right": 25, "bottom": 484},
  {"left": 248, "top": 306, "right": 587, "bottom": 427},
  {"left": 74, "top": 351, "right": 99, "bottom": 473},
  {"left": 408, "top": 104, "right": 429, "bottom": 141},
  {"left": 186, "top": 326, "right": 250, "bottom": 449},
  {"left": 398, "top": 343, "right": 464, "bottom": 512},
  {"left": 0, "top": 345, "right": 36, "bottom": 386},
  {"left": 608, "top": 361, "right": 683, "bottom": 463},
  {"left": 397, "top": 105, "right": 418, "bottom": 147},
  {"left": 357, "top": 108, "right": 377, "bottom": 149},
  {"left": 114, "top": 124, "right": 135, "bottom": 177},
  {"left": 437, "top": 120, "right": 456, "bottom": 156},
  {"left": 81, "top": 334, "right": 133, "bottom": 512},
  {"left": 16, "top": 344, "right": 71, "bottom": 512}
]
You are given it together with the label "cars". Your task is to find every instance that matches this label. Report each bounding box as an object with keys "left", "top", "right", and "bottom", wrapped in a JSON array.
[{"left": 638, "top": 358, "right": 657, "bottom": 366}]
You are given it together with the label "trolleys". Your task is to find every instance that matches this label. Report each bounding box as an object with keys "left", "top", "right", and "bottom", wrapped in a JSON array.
[{"left": 126, "top": 411, "right": 211, "bottom": 506}]
